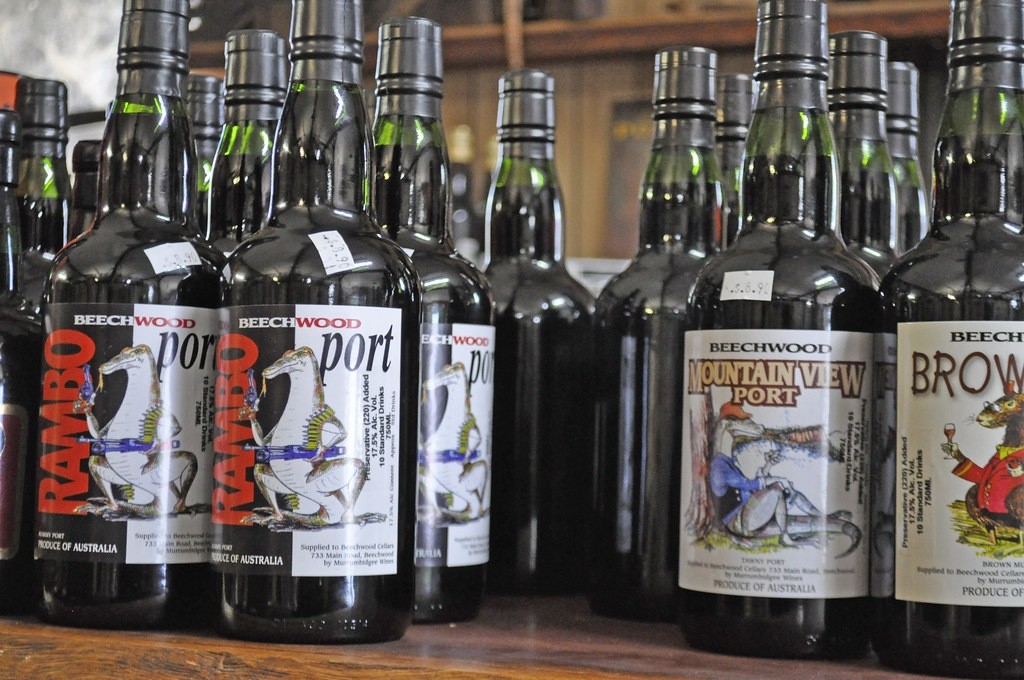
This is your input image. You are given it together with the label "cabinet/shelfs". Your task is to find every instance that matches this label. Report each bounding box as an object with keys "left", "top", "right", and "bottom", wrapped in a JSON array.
[{"left": 187, "top": 0, "right": 951, "bottom": 76}]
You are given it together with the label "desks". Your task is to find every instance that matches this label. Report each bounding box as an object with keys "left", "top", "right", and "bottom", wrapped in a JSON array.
[{"left": 0, "top": 591, "right": 952, "bottom": 679}]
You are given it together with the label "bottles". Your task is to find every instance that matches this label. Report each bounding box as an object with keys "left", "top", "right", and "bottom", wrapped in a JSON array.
[{"left": 0, "top": 0, "right": 1024, "bottom": 679}]
